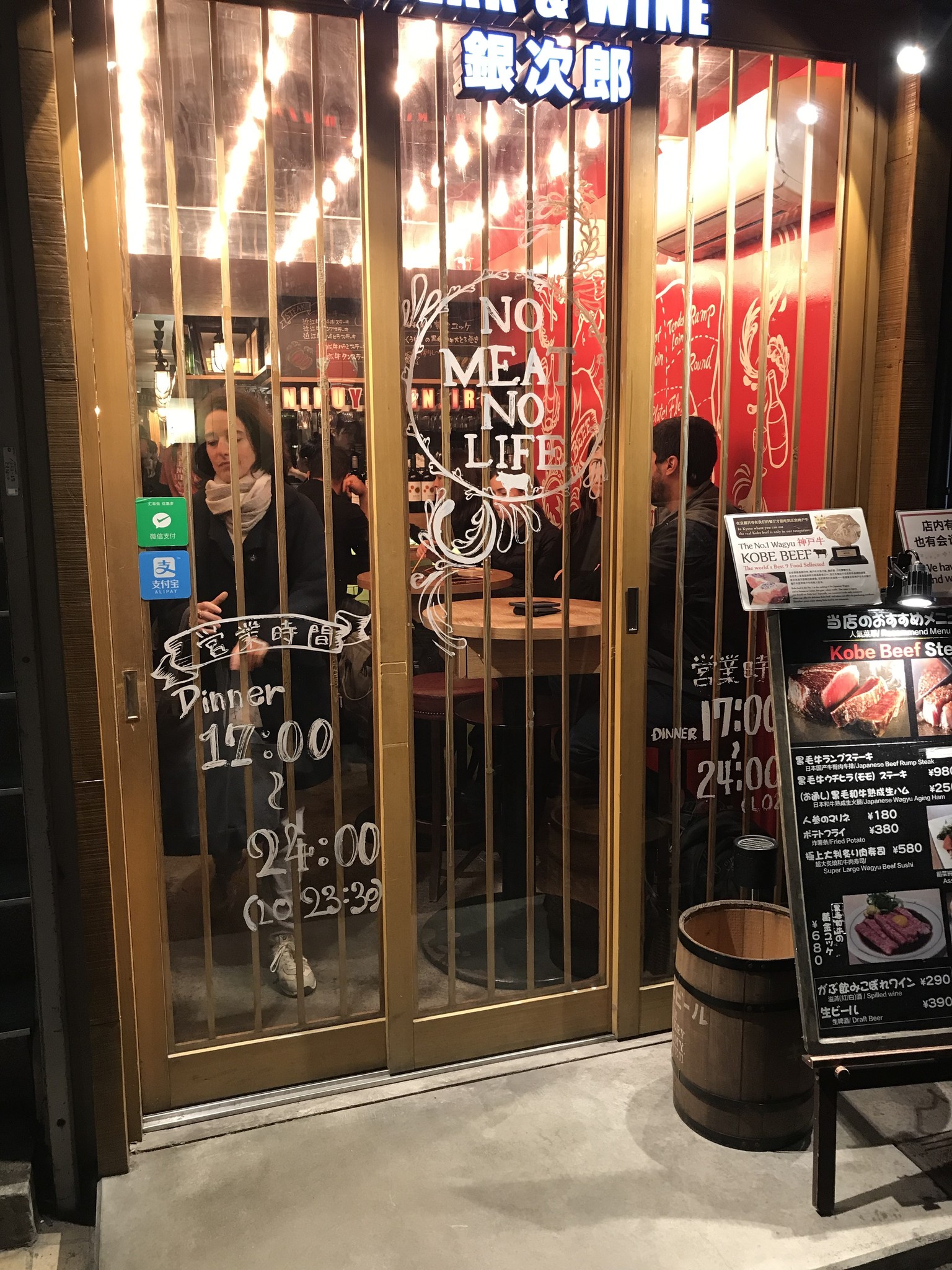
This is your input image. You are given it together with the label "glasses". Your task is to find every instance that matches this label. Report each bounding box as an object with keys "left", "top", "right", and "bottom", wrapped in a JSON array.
[{"left": 140, "top": 437, "right": 151, "bottom": 445}]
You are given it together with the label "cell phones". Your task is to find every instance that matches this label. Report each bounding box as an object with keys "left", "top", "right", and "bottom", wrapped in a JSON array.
[{"left": 509, "top": 600, "right": 561, "bottom": 608}]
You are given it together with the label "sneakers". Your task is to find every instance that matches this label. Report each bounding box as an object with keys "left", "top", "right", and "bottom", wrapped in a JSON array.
[
  {"left": 209, "top": 856, "right": 247, "bottom": 909},
  {"left": 267, "top": 931, "right": 317, "bottom": 998}
]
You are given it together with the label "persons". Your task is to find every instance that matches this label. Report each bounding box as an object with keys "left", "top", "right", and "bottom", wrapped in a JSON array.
[
  {"left": 554, "top": 416, "right": 749, "bottom": 855},
  {"left": 139, "top": 417, "right": 369, "bottom": 677},
  {"left": 409, "top": 448, "right": 492, "bottom": 550},
  {"left": 533, "top": 436, "right": 604, "bottom": 601},
  {"left": 416, "top": 463, "right": 562, "bottom": 599},
  {"left": 154, "top": 387, "right": 340, "bottom": 997}
]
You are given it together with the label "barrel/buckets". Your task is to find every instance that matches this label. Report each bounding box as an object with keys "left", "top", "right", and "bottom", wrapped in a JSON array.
[{"left": 672, "top": 900, "right": 814, "bottom": 1152}]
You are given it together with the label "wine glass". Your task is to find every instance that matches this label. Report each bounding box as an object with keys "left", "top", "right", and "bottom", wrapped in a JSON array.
[{"left": 235, "top": 385, "right": 477, "bottom": 433}]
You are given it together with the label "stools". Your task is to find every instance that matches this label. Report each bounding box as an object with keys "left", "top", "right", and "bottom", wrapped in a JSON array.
[
  {"left": 645, "top": 715, "right": 740, "bottom": 975},
  {"left": 411, "top": 674, "right": 500, "bottom": 899}
]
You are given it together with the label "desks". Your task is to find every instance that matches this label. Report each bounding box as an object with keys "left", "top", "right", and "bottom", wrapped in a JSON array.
[
  {"left": 346, "top": 562, "right": 513, "bottom": 848},
  {"left": 403, "top": 593, "right": 604, "bottom": 988}
]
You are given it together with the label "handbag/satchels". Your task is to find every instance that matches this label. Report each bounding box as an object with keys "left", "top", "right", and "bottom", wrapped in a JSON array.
[{"left": 156, "top": 606, "right": 284, "bottom": 856}]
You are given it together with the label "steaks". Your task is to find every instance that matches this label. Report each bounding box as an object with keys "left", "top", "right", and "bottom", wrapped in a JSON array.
[
  {"left": 915, "top": 657, "right": 952, "bottom": 732},
  {"left": 854, "top": 906, "right": 932, "bottom": 956},
  {"left": 787, "top": 663, "right": 906, "bottom": 737}
]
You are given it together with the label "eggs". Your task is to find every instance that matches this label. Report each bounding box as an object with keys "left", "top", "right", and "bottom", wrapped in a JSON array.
[{"left": 892, "top": 914, "right": 908, "bottom": 927}]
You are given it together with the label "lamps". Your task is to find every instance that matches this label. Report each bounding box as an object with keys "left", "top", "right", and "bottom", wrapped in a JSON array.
[
  {"left": 886, "top": 544, "right": 937, "bottom": 610},
  {"left": 210, "top": 330, "right": 235, "bottom": 373},
  {"left": 153, "top": 320, "right": 174, "bottom": 419}
]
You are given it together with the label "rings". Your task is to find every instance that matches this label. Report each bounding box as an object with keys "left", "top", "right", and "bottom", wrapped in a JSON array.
[{"left": 197, "top": 610, "right": 202, "bottom": 619}]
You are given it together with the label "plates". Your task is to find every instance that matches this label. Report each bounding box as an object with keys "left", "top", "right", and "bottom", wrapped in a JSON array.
[{"left": 845, "top": 898, "right": 946, "bottom": 963}]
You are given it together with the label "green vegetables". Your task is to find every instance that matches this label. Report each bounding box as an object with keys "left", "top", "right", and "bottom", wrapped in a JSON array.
[
  {"left": 865, "top": 891, "right": 899, "bottom": 914},
  {"left": 936, "top": 824, "right": 952, "bottom": 841}
]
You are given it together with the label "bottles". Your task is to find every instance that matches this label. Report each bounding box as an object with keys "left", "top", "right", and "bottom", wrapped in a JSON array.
[
  {"left": 183, "top": 322, "right": 195, "bottom": 375},
  {"left": 349, "top": 445, "right": 515, "bottom": 511}
]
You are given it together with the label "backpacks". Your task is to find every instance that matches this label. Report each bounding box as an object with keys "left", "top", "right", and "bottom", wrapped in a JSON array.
[{"left": 644, "top": 797, "right": 787, "bottom": 976}]
